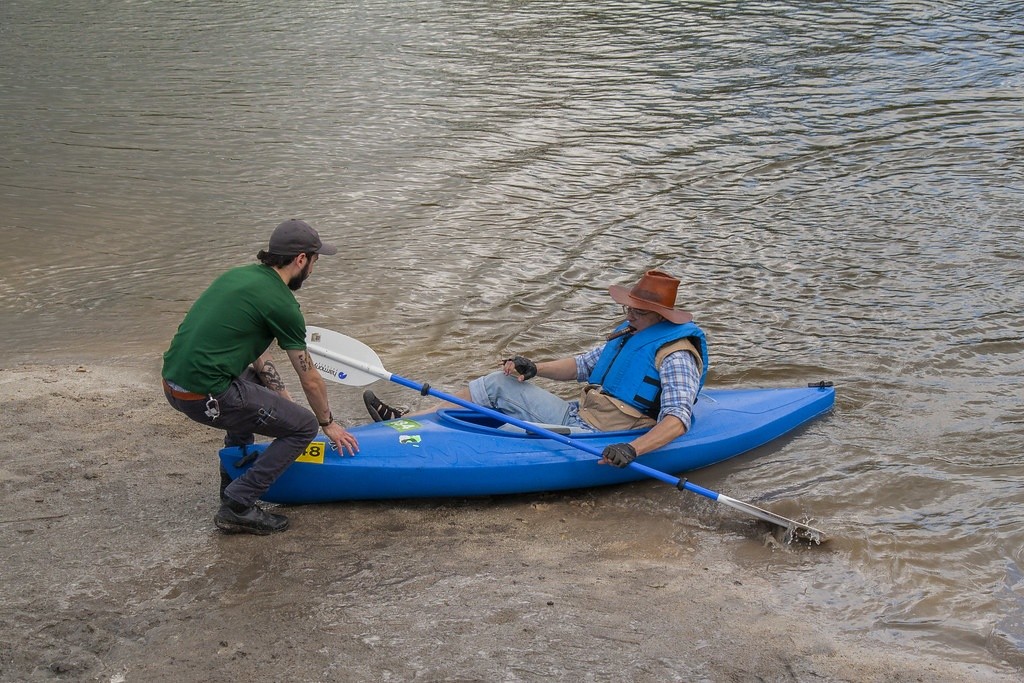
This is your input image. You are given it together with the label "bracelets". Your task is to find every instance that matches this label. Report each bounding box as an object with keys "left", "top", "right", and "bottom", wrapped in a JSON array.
[{"left": 319, "top": 412, "right": 333, "bottom": 426}]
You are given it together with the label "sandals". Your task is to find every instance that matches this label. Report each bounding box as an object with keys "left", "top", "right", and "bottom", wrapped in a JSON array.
[{"left": 362, "top": 389, "right": 402, "bottom": 422}]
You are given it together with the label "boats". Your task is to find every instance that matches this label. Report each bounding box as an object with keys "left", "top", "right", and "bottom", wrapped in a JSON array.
[{"left": 218, "top": 382, "right": 835, "bottom": 498}]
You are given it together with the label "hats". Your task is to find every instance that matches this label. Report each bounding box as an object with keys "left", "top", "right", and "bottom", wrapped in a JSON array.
[
  {"left": 609, "top": 270, "right": 693, "bottom": 324},
  {"left": 269, "top": 219, "right": 337, "bottom": 256}
]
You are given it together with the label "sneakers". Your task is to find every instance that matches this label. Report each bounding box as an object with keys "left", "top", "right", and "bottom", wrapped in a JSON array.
[
  {"left": 218, "top": 484, "right": 231, "bottom": 499},
  {"left": 214, "top": 500, "right": 290, "bottom": 535}
]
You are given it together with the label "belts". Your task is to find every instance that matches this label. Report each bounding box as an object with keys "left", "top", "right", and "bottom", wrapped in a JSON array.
[{"left": 161, "top": 378, "right": 208, "bottom": 401}]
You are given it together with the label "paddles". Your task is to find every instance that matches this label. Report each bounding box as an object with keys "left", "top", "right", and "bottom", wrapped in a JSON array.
[{"left": 303, "top": 327, "right": 829, "bottom": 539}]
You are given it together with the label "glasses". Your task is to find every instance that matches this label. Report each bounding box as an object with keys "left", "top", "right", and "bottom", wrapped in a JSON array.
[{"left": 622, "top": 305, "right": 653, "bottom": 319}]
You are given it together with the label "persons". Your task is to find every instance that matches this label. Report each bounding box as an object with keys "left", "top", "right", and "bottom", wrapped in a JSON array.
[
  {"left": 363, "top": 268, "right": 703, "bottom": 468},
  {"left": 161, "top": 219, "right": 359, "bottom": 536}
]
